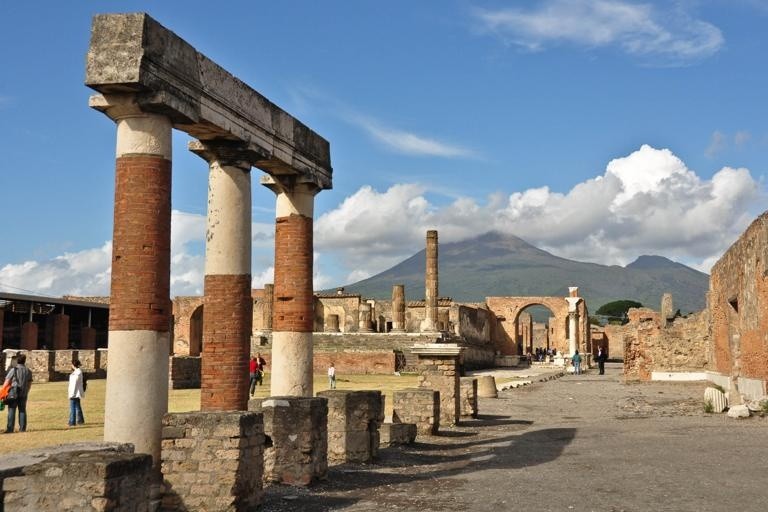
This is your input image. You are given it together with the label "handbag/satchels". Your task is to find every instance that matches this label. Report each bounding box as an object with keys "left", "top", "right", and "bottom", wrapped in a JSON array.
[
  {"left": 83, "top": 373, "right": 87, "bottom": 392},
  {"left": 1, "top": 383, "right": 21, "bottom": 403}
]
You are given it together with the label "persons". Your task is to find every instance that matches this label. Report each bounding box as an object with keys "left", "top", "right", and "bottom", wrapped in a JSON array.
[
  {"left": 597, "top": 344, "right": 605, "bottom": 375},
  {"left": 1, "top": 354, "right": 33, "bottom": 432},
  {"left": 67, "top": 358, "right": 88, "bottom": 426},
  {"left": 535, "top": 347, "right": 556, "bottom": 362},
  {"left": 249, "top": 356, "right": 257, "bottom": 397},
  {"left": 328, "top": 362, "right": 336, "bottom": 390},
  {"left": 571, "top": 349, "right": 582, "bottom": 376},
  {"left": 254, "top": 352, "right": 266, "bottom": 386},
  {"left": 526, "top": 350, "right": 532, "bottom": 366}
]
